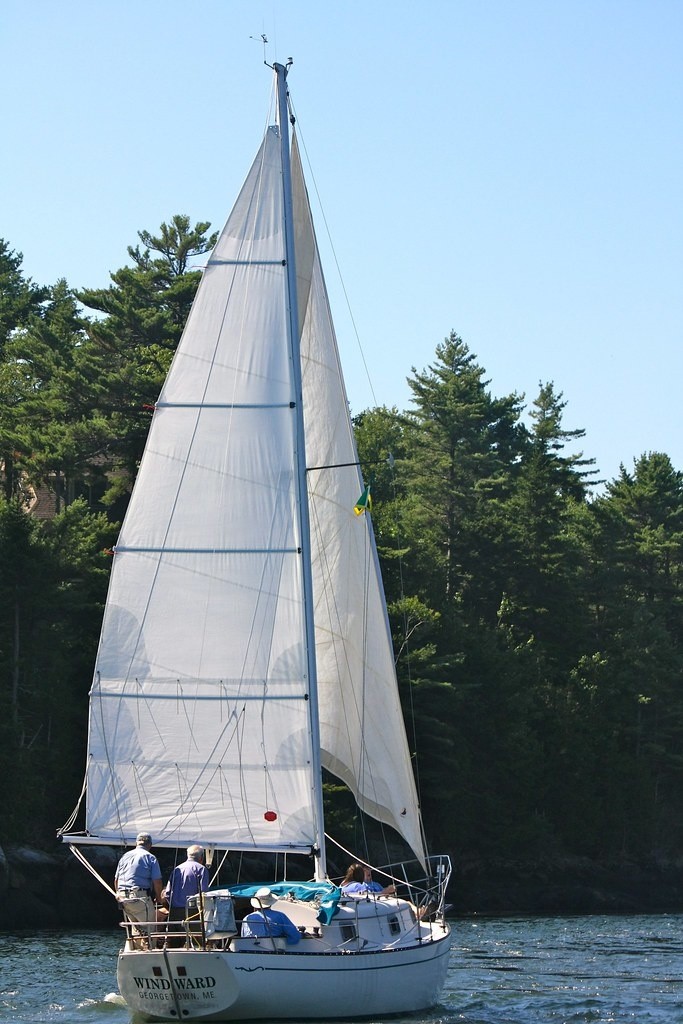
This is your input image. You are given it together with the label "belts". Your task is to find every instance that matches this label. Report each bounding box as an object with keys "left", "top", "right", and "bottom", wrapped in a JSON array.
[{"left": 120, "top": 888, "right": 149, "bottom": 892}]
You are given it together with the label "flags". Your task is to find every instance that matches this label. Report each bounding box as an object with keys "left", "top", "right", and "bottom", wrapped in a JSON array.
[{"left": 354, "top": 488, "right": 372, "bottom": 516}]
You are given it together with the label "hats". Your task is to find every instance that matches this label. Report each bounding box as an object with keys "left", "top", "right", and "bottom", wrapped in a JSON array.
[{"left": 250, "top": 887, "right": 278, "bottom": 908}]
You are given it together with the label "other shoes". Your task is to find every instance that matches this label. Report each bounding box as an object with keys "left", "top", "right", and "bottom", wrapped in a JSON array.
[{"left": 419, "top": 901, "right": 435, "bottom": 921}]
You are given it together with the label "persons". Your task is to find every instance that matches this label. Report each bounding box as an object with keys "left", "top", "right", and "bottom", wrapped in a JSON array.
[
  {"left": 241, "top": 888, "right": 300, "bottom": 945},
  {"left": 338, "top": 864, "right": 367, "bottom": 894},
  {"left": 163, "top": 845, "right": 209, "bottom": 948},
  {"left": 357, "top": 860, "right": 424, "bottom": 916},
  {"left": 115, "top": 833, "right": 162, "bottom": 947}
]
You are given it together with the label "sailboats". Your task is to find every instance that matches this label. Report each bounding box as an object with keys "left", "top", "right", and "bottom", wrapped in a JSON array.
[{"left": 53, "top": 33, "right": 452, "bottom": 1021}]
